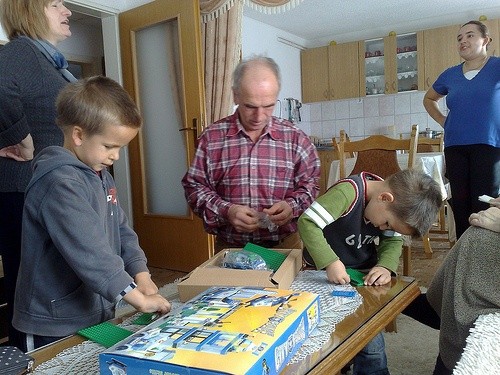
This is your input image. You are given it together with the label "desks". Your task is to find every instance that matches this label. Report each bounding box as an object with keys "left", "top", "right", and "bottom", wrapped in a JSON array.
[
  {"left": 327, "top": 152, "right": 445, "bottom": 257},
  {"left": 24, "top": 266, "right": 420, "bottom": 375}
]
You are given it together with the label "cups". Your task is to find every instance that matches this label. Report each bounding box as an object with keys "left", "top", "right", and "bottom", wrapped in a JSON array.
[{"left": 365, "top": 43, "right": 416, "bottom": 94}]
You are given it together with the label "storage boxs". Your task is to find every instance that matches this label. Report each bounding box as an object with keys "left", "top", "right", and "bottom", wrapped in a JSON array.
[
  {"left": 177, "top": 248, "right": 302, "bottom": 302},
  {"left": 99, "top": 286, "right": 320, "bottom": 375}
]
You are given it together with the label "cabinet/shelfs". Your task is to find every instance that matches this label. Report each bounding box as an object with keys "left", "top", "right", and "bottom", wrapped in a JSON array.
[
  {"left": 424, "top": 19, "right": 500, "bottom": 91},
  {"left": 359, "top": 30, "right": 424, "bottom": 97},
  {"left": 301, "top": 40, "right": 359, "bottom": 104}
]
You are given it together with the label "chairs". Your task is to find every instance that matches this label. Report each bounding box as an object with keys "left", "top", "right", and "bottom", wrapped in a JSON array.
[{"left": 333, "top": 129, "right": 445, "bottom": 259}]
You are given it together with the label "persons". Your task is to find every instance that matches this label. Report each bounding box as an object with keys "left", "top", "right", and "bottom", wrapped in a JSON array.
[
  {"left": 297, "top": 168, "right": 442, "bottom": 375},
  {"left": 12, "top": 77, "right": 170, "bottom": 353},
  {"left": 423, "top": 20, "right": 500, "bottom": 242},
  {"left": 399, "top": 194, "right": 500, "bottom": 375},
  {"left": 180, "top": 54, "right": 320, "bottom": 257},
  {"left": 0, "top": 0, "right": 79, "bottom": 309}
]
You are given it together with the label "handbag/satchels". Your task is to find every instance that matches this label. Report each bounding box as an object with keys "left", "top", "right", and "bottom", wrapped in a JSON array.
[{"left": 0, "top": 346, "right": 35, "bottom": 375}]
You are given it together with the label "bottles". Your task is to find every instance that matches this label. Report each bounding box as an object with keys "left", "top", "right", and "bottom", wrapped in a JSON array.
[
  {"left": 420, "top": 129, "right": 433, "bottom": 140},
  {"left": 309, "top": 135, "right": 323, "bottom": 147}
]
